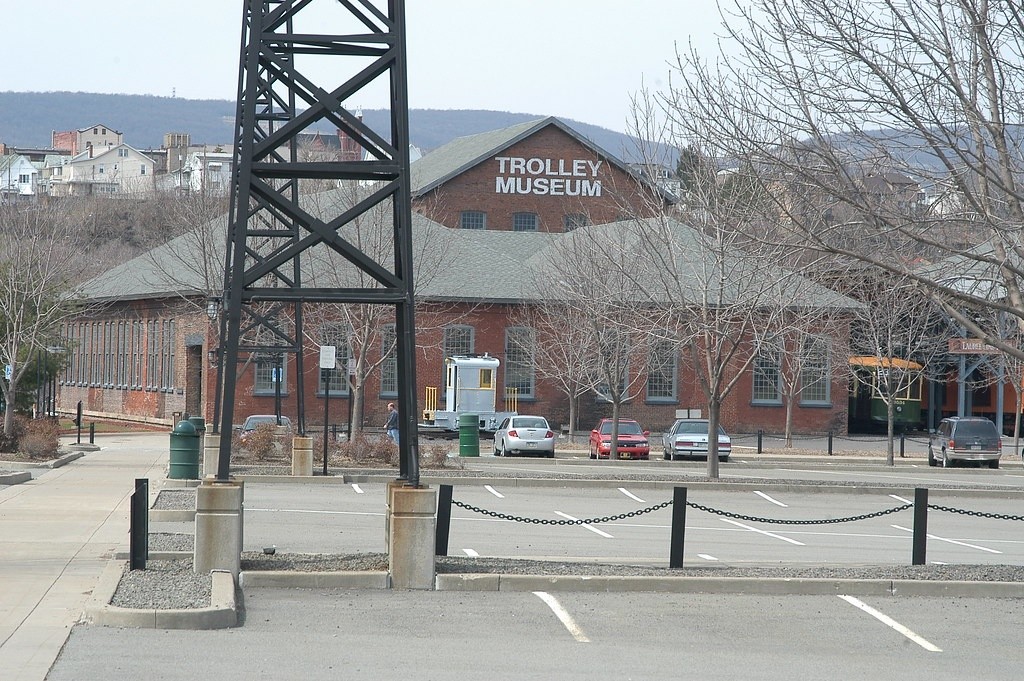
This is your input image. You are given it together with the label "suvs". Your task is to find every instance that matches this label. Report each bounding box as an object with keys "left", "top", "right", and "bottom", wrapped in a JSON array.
[{"left": 928, "top": 416, "right": 1002, "bottom": 470}]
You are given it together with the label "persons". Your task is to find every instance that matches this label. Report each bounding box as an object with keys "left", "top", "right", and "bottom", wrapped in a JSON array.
[{"left": 383, "top": 403, "right": 399, "bottom": 444}]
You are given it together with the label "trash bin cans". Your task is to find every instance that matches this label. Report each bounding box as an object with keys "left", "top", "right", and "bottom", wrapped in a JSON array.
[
  {"left": 188, "top": 417, "right": 206, "bottom": 430},
  {"left": 169, "top": 420, "right": 200, "bottom": 480},
  {"left": 458, "top": 412, "right": 480, "bottom": 457}
]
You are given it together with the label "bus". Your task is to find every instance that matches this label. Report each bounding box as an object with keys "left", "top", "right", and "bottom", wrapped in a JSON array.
[{"left": 847, "top": 353, "right": 923, "bottom": 429}]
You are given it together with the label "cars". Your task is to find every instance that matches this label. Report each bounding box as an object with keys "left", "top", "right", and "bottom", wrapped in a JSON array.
[
  {"left": 662, "top": 419, "right": 732, "bottom": 463},
  {"left": 236, "top": 415, "right": 293, "bottom": 444},
  {"left": 589, "top": 417, "right": 650, "bottom": 460},
  {"left": 493, "top": 415, "right": 555, "bottom": 458}
]
